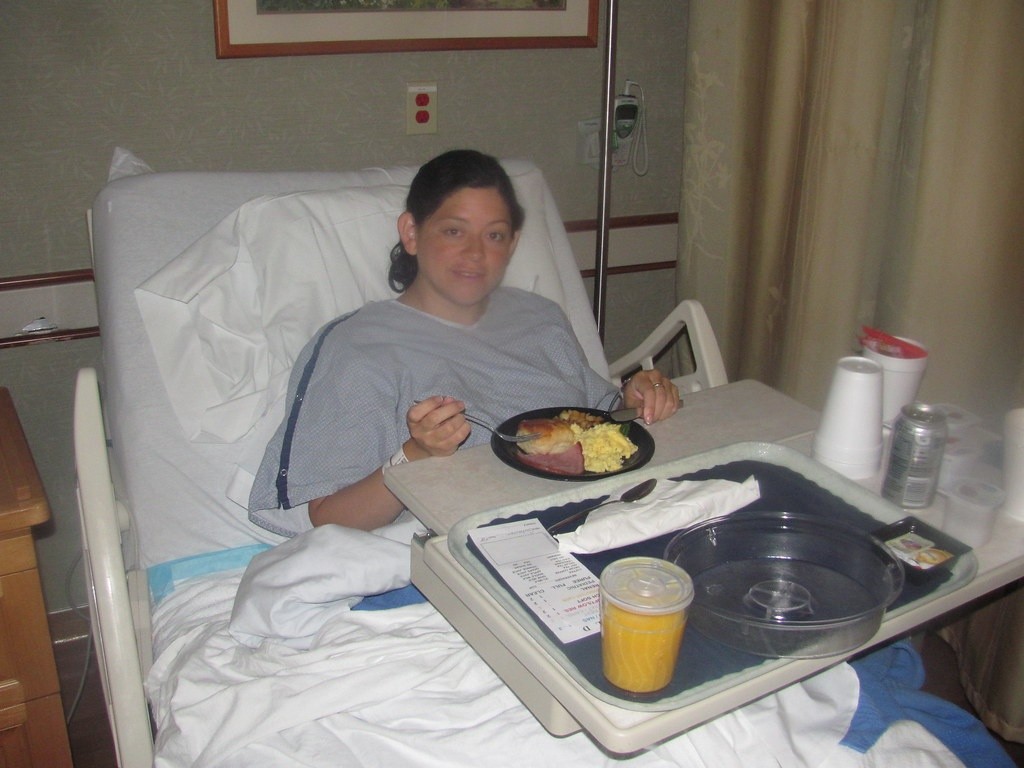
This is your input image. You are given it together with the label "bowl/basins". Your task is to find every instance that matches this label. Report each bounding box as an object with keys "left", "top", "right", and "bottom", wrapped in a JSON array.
[{"left": 870, "top": 516, "right": 973, "bottom": 586}]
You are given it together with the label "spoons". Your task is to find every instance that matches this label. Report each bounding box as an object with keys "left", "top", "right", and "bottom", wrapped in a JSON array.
[{"left": 548, "top": 478, "right": 657, "bottom": 536}]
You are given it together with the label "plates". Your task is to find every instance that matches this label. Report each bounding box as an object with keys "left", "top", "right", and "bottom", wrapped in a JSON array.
[
  {"left": 664, "top": 511, "right": 905, "bottom": 659},
  {"left": 491, "top": 406, "right": 655, "bottom": 482}
]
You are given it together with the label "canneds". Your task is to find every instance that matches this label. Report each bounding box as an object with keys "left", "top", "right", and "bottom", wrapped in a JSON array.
[{"left": 881, "top": 403, "right": 947, "bottom": 509}]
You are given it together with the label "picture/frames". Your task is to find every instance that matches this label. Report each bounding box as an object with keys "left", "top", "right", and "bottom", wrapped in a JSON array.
[{"left": 213, "top": 0, "right": 599, "bottom": 58}]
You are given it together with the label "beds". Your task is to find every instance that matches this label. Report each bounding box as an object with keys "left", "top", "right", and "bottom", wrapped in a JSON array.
[{"left": 73, "top": 157, "right": 1016, "bottom": 767}]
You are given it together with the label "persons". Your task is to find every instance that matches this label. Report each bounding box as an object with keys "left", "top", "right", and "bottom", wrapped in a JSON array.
[{"left": 247, "top": 145, "right": 679, "bottom": 538}]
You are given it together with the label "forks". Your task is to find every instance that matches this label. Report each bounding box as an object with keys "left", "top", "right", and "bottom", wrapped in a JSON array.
[{"left": 414, "top": 400, "right": 544, "bottom": 442}]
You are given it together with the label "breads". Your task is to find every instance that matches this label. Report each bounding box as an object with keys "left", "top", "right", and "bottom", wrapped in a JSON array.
[{"left": 516, "top": 417, "right": 575, "bottom": 455}]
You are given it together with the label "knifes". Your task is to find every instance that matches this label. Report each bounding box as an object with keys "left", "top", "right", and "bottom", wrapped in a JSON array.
[{"left": 610, "top": 399, "right": 684, "bottom": 421}]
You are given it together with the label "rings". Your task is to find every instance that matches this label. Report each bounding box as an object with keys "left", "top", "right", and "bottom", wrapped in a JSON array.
[{"left": 653, "top": 383, "right": 663, "bottom": 387}]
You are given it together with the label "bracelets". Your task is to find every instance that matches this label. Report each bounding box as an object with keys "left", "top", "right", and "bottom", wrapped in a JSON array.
[
  {"left": 382, "top": 455, "right": 393, "bottom": 477},
  {"left": 391, "top": 445, "right": 409, "bottom": 467}
]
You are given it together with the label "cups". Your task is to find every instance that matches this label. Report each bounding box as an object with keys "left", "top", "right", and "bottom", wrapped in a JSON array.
[
  {"left": 599, "top": 556, "right": 694, "bottom": 693},
  {"left": 1004, "top": 407, "right": 1023, "bottom": 522},
  {"left": 863, "top": 336, "right": 929, "bottom": 424},
  {"left": 944, "top": 478, "right": 1005, "bottom": 549},
  {"left": 812, "top": 356, "right": 884, "bottom": 479}
]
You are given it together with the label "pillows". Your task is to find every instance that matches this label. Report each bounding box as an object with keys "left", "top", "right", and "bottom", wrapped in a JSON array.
[{"left": 133, "top": 192, "right": 411, "bottom": 452}]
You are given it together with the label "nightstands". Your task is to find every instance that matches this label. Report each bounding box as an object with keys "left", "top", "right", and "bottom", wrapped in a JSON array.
[{"left": 1, "top": 388, "right": 76, "bottom": 768}]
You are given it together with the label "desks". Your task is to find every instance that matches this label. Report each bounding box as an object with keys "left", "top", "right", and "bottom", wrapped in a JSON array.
[{"left": 383, "top": 377, "right": 1023, "bottom": 752}]
